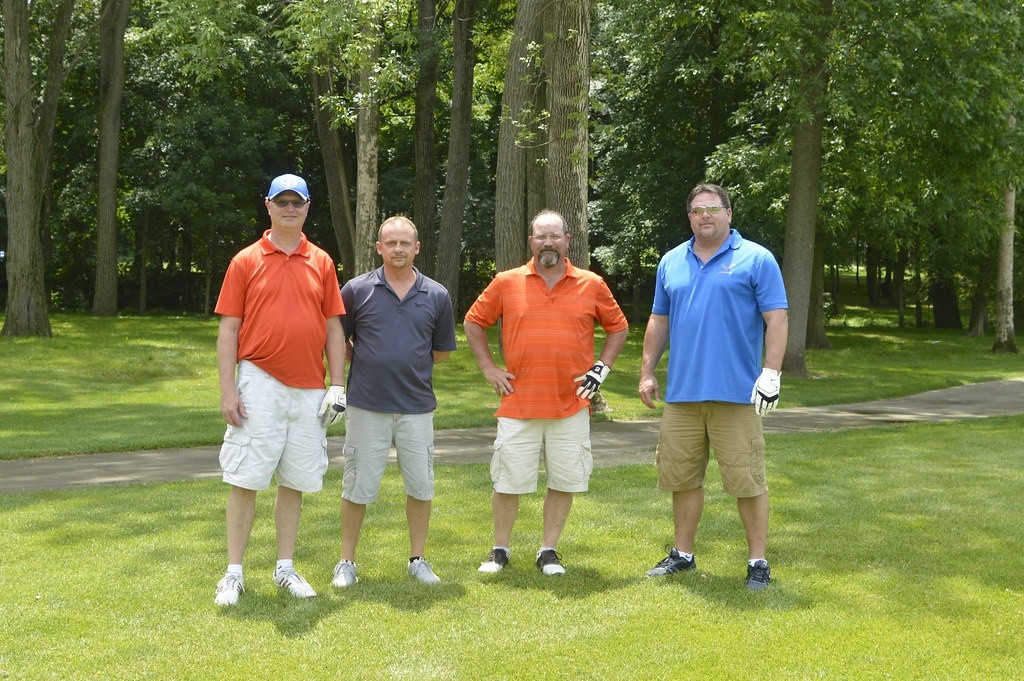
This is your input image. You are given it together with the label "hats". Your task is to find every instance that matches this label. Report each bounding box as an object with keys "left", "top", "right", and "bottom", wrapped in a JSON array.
[{"left": 268, "top": 174, "right": 309, "bottom": 202}]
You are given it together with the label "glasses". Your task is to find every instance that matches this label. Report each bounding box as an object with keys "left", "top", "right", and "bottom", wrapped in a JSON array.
[
  {"left": 691, "top": 205, "right": 727, "bottom": 216},
  {"left": 268, "top": 199, "right": 309, "bottom": 209}
]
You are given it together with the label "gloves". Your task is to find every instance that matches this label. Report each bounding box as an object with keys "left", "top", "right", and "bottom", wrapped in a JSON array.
[
  {"left": 572, "top": 359, "right": 611, "bottom": 400},
  {"left": 750, "top": 368, "right": 782, "bottom": 416},
  {"left": 318, "top": 384, "right": 346, "bottom": 427}
]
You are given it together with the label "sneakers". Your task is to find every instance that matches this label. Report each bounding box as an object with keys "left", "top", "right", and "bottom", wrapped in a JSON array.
[
  {"left": 214, "top": 571, "right": 246, "bottom": 606},
  {"left": 744, "top": 560, "right": 771, "bottom": 590},
  {"left": 477, "top": 548, "right": 510, "bottom": 573},
  {"left": 272, "top": 567, "right": 318, "bottom": 597},
  {"left": 332, "top": 563, "right": 359, "bottom": 587},
  {"left": 408, "top": 557, "right": 441, "bottom": 585},
  {"left": 644, "top": 544, "right": 696, "bottom": 578},
  {"left": 536, "top": 549, "right": 566, "bottom": 576}
]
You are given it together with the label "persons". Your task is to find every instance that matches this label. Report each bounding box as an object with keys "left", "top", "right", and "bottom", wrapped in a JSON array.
[
  {"left": 463, "top": 210, "right": 630, "bottom": 576},
  {"left": 214, "top": 173, "right": 347, "bottom": 607},
  {"left": 331, "top": 215, "right": 457, "bottom": 588},
  {"left": 638, "top": 182, "right": 789, "bottom": 592}
]
